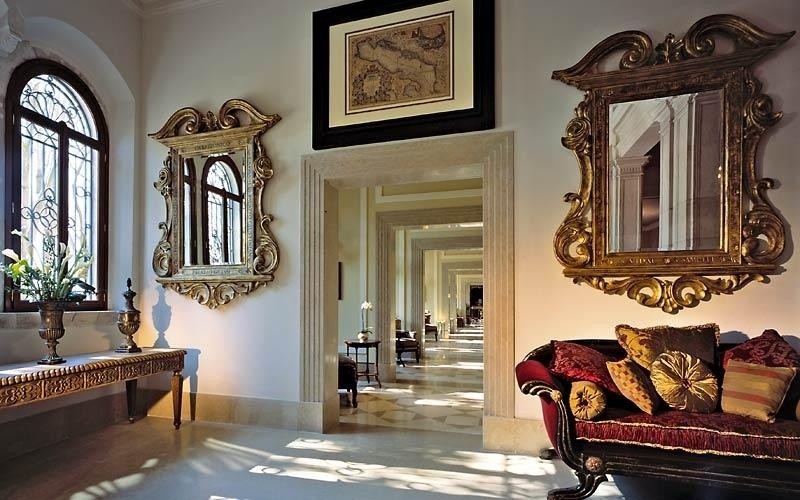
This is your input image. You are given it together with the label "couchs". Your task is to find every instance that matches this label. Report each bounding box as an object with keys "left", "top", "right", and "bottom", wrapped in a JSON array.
[
  {"left": 395, "top": 330, "right": 421, "bottom": 363},
  {"left": 515, "top": 339, "right": 800, "bottom": 500},
  {"left": 338, "top": 353, "right": 358, "bottom": 408}
]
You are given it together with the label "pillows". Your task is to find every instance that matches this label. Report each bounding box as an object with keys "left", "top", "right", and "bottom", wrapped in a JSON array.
[{"left": 546, "top": 324, "right": 799, "bottom": 423}]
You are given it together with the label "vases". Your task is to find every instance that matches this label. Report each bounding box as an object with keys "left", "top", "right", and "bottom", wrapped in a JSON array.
[
  {"left": 36, "top": 300, "right": 68, "bottom": 364},
  {"left": 358, "top": 333, "right": 368, "bottom": 343}
]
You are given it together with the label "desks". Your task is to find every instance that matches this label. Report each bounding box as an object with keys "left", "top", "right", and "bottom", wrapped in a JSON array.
[{"left": 0, "top": 347, "right": 187, "bottom": 429}]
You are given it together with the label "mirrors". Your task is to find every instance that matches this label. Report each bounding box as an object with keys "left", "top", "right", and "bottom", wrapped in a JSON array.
[
  {"left": 147, "top": 98, "right": 280, "bottom": 309},
  {"left": 551, "top": 14, "right": 796, "bottom": 314}
]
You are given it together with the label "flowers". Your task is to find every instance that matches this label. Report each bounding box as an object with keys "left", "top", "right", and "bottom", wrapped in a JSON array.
[
  {"left": 357, "top": 301, "right": 373, "bottom": 333},
  {"left": 0, "top": 225, "right": 95, "bottom": 307}
]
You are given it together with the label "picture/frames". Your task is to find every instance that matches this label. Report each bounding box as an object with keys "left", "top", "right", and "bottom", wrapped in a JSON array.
[{"left": 312, "top": 0, "right": 495, "bottom": 150}]
